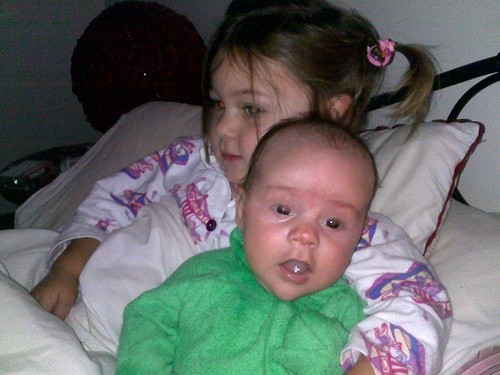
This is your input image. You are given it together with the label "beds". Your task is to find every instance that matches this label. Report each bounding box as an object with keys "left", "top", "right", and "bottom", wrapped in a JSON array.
[{"left": 0, "top": 52, "right": 500, "bottom": 375}]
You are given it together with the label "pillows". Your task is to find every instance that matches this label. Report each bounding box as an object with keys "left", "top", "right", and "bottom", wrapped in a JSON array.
[{"left": 14, "top": 102, "right": 486, "bottom": 257}]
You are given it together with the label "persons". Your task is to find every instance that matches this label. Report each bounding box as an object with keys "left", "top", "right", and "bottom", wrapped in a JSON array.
[
  {"left": 29, "top": 0, "right": 454, "bottom": 375},
  {"left": 114, "top": 107, "right": 378, "bottom": 375},
  {"left": 69, "top": 1, "right": 209, "bottom": 136}
]
